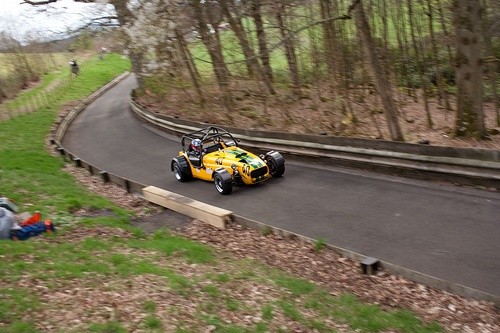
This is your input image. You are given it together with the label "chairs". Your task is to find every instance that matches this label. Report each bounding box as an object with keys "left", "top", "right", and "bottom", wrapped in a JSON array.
[{"left": 203, "top": 142, "right": 224, "bottom": 153}]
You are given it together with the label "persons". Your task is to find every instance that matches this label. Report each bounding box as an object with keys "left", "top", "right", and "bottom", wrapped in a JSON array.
[{"left": 189, "top": 139, "right": 210, "bottom": 158}]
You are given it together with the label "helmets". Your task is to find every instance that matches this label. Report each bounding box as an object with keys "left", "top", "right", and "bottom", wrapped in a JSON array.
[{"left": 189, "top": 139, "right": 202, "bottom": 151}]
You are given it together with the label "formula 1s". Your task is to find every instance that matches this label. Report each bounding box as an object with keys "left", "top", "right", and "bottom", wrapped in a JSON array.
[{"left": 171, "top": 126, "right": 286, "bottom": 195}]
